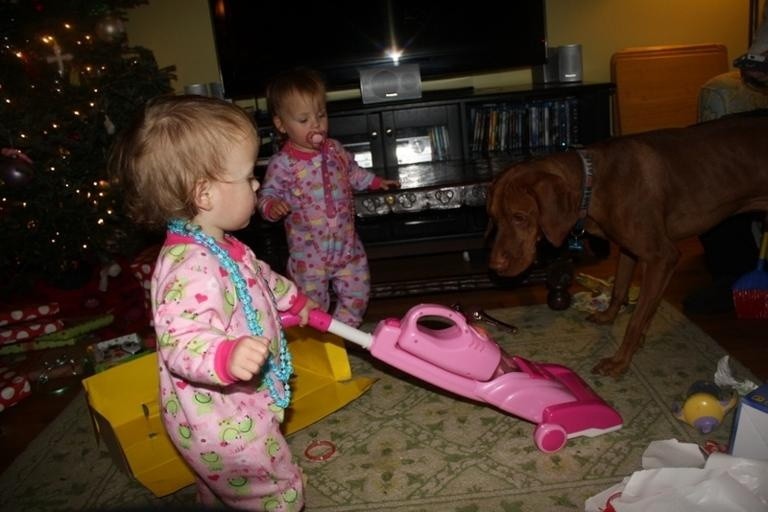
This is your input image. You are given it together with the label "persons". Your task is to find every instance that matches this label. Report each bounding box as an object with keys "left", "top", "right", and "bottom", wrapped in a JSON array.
[
  {"left": 254, "top": 72, "right": 402, "bottom": 325},
  {"left": 112, "top": 94, "right": 322, "bottom": 512}
]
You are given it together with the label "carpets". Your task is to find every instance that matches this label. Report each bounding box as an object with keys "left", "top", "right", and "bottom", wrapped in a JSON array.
[{"left": 2, "top": 286, "right": 766, "bottom": 510}]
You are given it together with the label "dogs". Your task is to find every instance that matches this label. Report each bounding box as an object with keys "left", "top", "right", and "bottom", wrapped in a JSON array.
[{"left": 481, "top": 106, "right": 767, "bottom": 382}]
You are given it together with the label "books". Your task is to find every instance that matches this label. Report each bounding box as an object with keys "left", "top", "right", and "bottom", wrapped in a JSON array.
[
  {"left": 469, "top": 98, "right": 582, "bottom": 150},
  {"left": 429, "top": 123, "right": 452, "bottom": 163}
]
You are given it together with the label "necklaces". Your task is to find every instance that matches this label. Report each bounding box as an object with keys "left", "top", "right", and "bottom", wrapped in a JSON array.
[{"left": 165, "top": 218, "right": 296, "bottom": 411}]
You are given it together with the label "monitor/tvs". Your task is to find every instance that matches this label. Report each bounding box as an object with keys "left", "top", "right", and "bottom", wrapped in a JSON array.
[{"left": 208, "top": 0, "right": 548, "bottom": 100}]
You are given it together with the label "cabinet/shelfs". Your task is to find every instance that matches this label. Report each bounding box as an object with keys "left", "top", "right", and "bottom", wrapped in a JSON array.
[{"left": 234, "top": 82, "right": 618, "bottom": 312}]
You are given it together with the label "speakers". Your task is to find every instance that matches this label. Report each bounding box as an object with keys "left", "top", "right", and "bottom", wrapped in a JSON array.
[
  {"left": 185, "top": 83, "right": 225, "bottom": 100},
  {"left": 360, "top": 64, "right": 423, "bottom": 104},
  {"left": 545, "top": 45, "right": 583, "bottom": 82}
]
